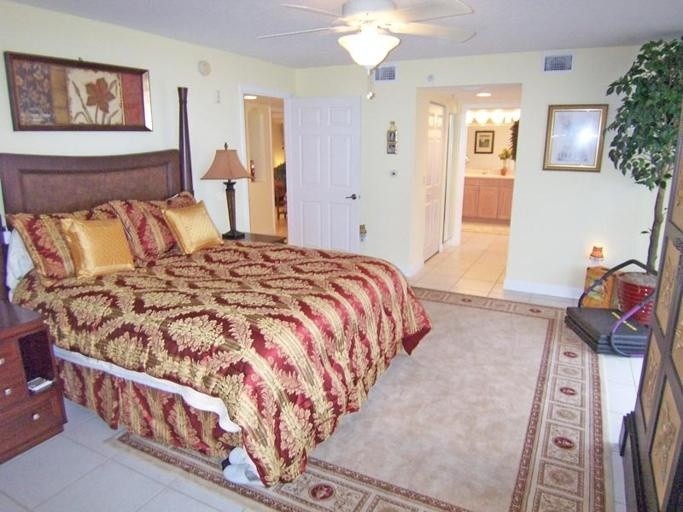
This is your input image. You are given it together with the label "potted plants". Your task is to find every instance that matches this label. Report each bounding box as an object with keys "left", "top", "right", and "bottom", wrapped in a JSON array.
[
  {"left": 602, "top": 39, "right": 682, "bottom": 328},
  {"left": 498, "top": 148, "right": 510, "bottom": 176}
]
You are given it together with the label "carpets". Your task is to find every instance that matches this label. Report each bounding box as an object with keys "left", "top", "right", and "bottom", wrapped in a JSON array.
[{"left": 116, "top": 286, "right": 602, "bottom": 512}]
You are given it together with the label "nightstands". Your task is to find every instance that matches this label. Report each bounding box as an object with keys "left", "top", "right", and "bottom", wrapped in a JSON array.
[{"left": 0, "top": 296, "right": 67, "bottom": 463}]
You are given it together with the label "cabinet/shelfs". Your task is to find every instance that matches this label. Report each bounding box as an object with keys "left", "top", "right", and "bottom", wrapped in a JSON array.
[{"left": 462, "top": 177, "right": 513, "bottom": 223}]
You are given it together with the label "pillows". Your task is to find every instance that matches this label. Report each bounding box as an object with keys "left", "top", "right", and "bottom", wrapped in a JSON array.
[{"left": 3, "top": 186, "right": 221, "bottom": 287}]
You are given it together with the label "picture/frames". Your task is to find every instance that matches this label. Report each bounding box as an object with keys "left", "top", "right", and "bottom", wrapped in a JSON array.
[
  {"left": 473, "top": 130, "right": 494, "bottom": 154},
  {"left": 0, "top": 47, "right": 154, "bottom": 133},
  {"left": 543, "top": 104, "right": 609, "bottom": 173}
]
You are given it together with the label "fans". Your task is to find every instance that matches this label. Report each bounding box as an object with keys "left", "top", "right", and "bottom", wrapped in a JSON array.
[{"left": 258, "top": 0, "right": 476, "bottom": 75}]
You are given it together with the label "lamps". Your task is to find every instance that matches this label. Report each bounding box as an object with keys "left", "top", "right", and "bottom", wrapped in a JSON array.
[
  {"left": 200, "top": 141, "right": 254, "bottom": 241},
  {"left": 338, "top": 25, "right": 399, "bottom": 101}
]
